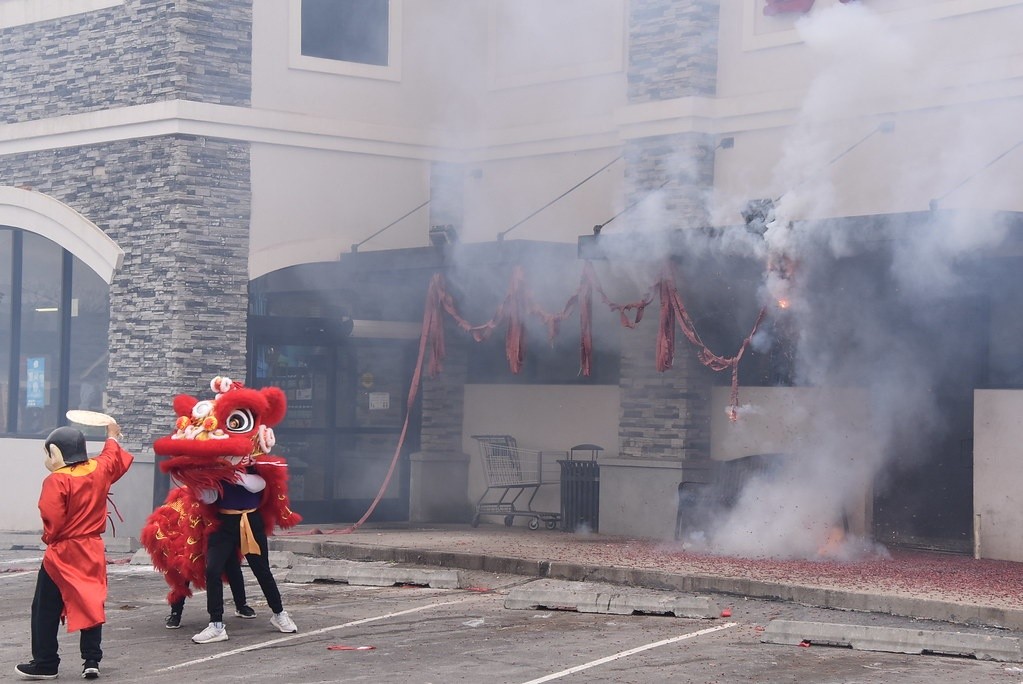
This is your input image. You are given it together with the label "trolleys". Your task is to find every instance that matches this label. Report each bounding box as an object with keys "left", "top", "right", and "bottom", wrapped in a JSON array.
[{"left": 465, "top": 435, "right": 562, "bottom": 531}]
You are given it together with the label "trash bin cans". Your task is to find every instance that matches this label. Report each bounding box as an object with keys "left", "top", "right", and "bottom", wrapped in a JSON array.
[{"left": 556, "top": 444, "right": 605, "bottom": 528}]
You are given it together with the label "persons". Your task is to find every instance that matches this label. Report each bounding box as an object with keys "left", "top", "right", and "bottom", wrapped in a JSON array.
[
  {"left": 192, "top": 464, "right": 298, "bottom": 645},
  {"left": 13, "top": 423, "right": 134, "bottom": 679},
  {"left": 164, "top": 561, "right": 257, "bottom": 628}
]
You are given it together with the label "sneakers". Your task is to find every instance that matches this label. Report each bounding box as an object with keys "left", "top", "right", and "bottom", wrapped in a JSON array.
[
  {"left": 192, "top": 623, "right": 228, "bottom": 643},
  {"left": 15, "top": 658, "right": 100, "bottom": 680},
  {"left": 270, "top": 611, "right": 297, "bottom": 634},
  {"left": 165, "top": 612, "right": 182, "bottom": 628},
  {"left": 235, "top": 606, "right": 256, "bottom": 619}
]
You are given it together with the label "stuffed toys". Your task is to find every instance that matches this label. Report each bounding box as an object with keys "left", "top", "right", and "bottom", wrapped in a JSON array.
[{"left": 138, "top": 373, "right": 303, "bottom": 605}]
